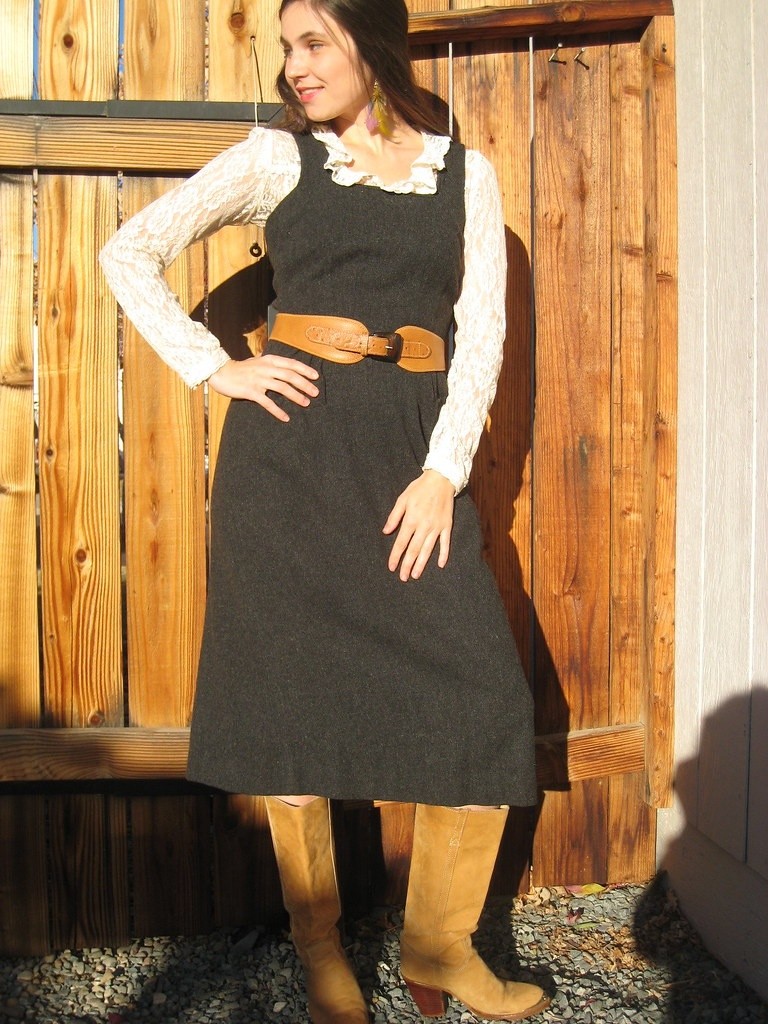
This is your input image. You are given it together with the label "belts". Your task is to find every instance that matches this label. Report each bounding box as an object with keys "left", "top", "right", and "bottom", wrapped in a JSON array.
[{"left": 266, "top": 313, "right": 447, "bottom": 375}]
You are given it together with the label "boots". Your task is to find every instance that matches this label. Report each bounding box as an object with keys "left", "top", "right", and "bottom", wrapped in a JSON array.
[
  {"left": 398, "top": 805, "right": 550, "bottom": 1019},
  {"left": 258, "top": 797, "right": 368, "bottom": 1024}
]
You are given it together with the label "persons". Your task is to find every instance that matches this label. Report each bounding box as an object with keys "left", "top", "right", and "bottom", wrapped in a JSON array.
[{"left": 98, "top": 0, "right": 551, "bottom": 1023}]
